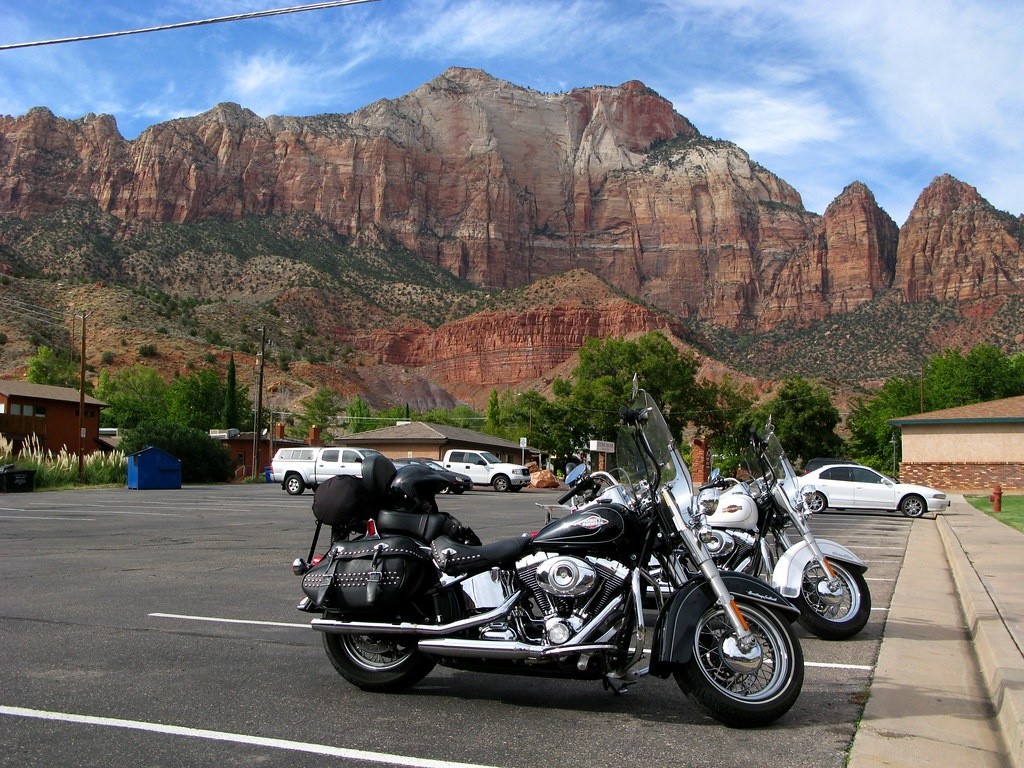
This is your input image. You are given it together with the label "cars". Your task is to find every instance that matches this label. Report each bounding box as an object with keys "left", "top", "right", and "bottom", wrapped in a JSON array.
[
  {"left": 389, "top": 458, "right": 474, "bottom": 495},
  {"left": 772, "top": 464, "right": 952, "bottom": 517},
  {"left": 804, "top": 456, "right": 856, "bottom": 475}
]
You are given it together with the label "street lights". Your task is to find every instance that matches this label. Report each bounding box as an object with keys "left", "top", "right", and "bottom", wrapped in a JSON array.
[
  {"left": 888, "top": 432, "right": 897, "bottom": 475},
  {"left": 73, "top": 309, "right": 99, "bottom": 483}
]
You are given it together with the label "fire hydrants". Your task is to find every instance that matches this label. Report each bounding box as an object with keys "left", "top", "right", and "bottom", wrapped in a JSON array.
[{"left": 989, "top": 483, "right": 1003, "bottom": 512}]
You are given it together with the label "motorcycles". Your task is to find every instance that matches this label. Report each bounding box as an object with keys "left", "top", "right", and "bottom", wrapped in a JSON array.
[
  {"left": 522, "top": 426, "right": 871, "bottom": 641},
  {"left": 292, "top": 373, "right": 804, "bottom": 729}
]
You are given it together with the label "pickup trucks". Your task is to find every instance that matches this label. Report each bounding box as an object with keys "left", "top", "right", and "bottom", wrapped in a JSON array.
[
  {"left": 269, "top": 446, "right": 411, "bottom": 495},
  {"left": 409, "top": 449, "right": 531, "bottom": 493}
]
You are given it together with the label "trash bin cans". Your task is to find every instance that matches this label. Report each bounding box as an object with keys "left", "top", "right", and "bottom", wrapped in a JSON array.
[
  {"left": 2, "top": 463, "right": 37, "bottom": 493},
  {"left": 127, "top": 445, "right": 184, "bottom": 490},
  {"left": 264, "top": 467, "right": 273, "bottom": 483}
]
